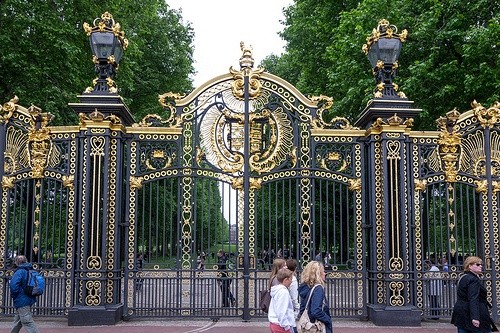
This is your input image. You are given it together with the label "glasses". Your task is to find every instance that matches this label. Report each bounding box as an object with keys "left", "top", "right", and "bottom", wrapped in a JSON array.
[{"left": 470, "top": 263, "right": 484, "bottom": 267}]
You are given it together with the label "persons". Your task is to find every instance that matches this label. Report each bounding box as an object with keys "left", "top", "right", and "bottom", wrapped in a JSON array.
[
  {"left": 217, "top": 249, "right": 236, "bottom": 307},
  {"left": 197, "top": 252, "right": 206, "bottom": 274},
  {"left": 316, "top": 252, "right": 321, "bottom": 260},
  {"left": 212, "top": 252, "right": 215, "bottom": 259},
  {"left": 268, "top": 268, "right": 297, "bottom": 333},
  {"left": 136, "top": 252, "right": 144, "bottom": 290},
  {"left": 286, "top": 259, "right": 301, "bottom": 333},
  {"left": 10, "top": 255, "right": 40, "bottom": 333},
  {"left": 297, "top": 260, "right": 333, "bottom": 333},
  {"left": 443, "top": 258, "right": 449, "bottom": 287},
  {"left": 450, "top": 256, "right": 492, "bottom": 333},
  {"left": 428, "top": 259, "right": 442, "bottom": 320},
  {"left": 268, "top": 259, "right": 286, "bottom": 286},
  {"left": 324, "top": 252, "right": 334, "bottom": 284},
  {"left": 257, "top": 248, "right": 282, "bottom": 270}
]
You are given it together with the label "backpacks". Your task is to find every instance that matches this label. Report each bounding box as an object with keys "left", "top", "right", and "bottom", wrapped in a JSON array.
[{"left": 15, "top": 268, "right": 44, "bottom": 297}]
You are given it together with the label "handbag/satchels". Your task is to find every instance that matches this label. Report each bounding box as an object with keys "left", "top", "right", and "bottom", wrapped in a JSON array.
[
  {"left": 297, "top": 310, "right": 326, "bottom": 333},
  {"left": 260, "top": 290, "right": 272, "bottom": 313}
]
